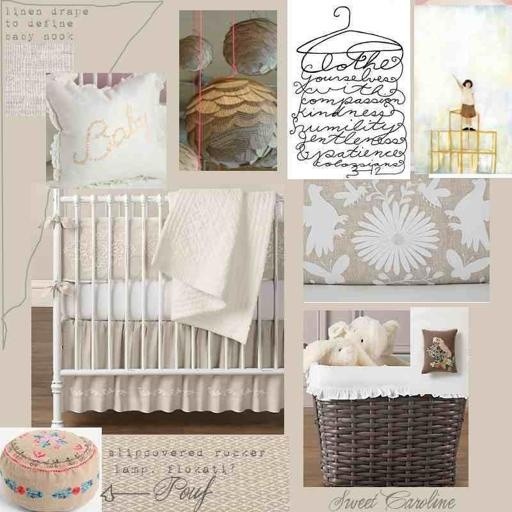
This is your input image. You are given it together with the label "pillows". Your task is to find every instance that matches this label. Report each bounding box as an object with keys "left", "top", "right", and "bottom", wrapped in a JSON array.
[
  {"left": 420, "top": 328, "right": 458, "bottom": 374},
  {"left": 0, "top": 429, "right": 97, "bottom": 511},
  {"left": 47, "top": 74, "right": 162, "bottom": 180}
]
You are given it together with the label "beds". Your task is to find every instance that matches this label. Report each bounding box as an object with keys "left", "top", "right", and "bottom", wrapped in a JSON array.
[{"left": 51, "top": 188, "right": 284, "bottom": 426}]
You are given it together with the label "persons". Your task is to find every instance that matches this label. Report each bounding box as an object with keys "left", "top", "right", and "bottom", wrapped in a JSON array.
[
  {"left": 302, "top": 337, "right": 376, "bottom": 376},
  {"left": 450, "top": 71, "right": 477, "bottom": 131}
]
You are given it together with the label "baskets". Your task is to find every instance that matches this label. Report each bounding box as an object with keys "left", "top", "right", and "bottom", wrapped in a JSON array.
[{"left": 312, "top": 361, "right": 465, "bottom": 485}]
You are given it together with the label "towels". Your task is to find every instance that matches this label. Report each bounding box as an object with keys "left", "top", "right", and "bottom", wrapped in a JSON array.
[{"left": 151, "top": 190, "right": 275, "bottom": 346}]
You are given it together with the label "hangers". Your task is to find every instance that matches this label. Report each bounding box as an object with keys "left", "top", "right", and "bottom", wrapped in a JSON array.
[{"left": 296, "top": 5, "right": 401, "bottom": 53}]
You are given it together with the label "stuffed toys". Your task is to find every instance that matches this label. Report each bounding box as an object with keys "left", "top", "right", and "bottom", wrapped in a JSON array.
[{"left": 326, "top": 315, "right": 400, "bottom": 366}]
